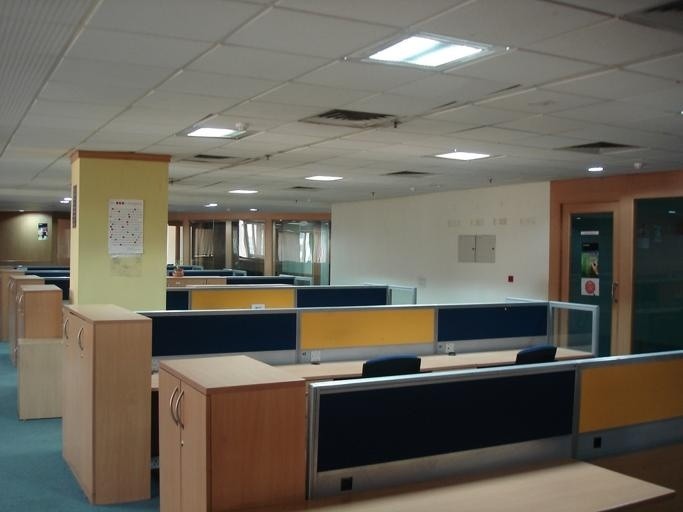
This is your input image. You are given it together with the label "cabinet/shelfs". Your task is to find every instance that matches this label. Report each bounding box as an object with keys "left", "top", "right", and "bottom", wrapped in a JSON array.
[{"left": 0, "top": 265, "right": 63, "bottom": 420}]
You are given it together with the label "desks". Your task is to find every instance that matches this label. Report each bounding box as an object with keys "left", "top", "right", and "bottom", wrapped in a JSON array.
[{"left": 310, "top": 453, "right": 674, "bottom": 511}]
